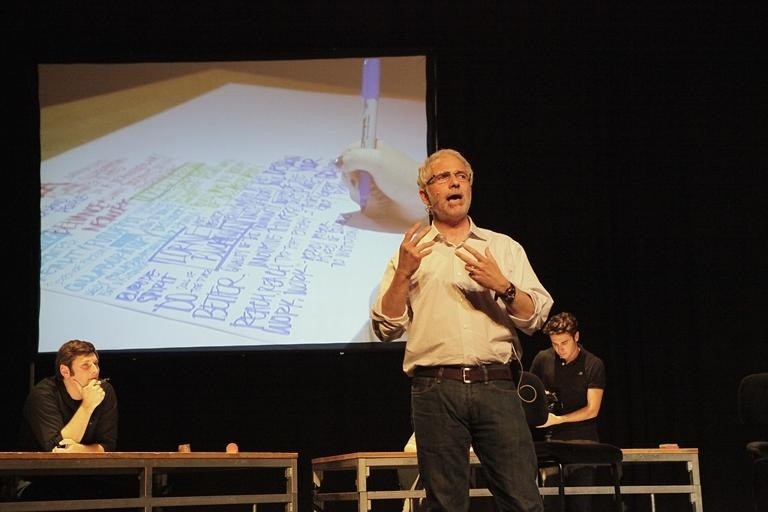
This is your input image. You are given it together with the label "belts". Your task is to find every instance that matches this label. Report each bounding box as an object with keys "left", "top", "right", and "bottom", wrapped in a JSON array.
[{"left": 414, "top": 366, "right": 513, "bottom": 384}]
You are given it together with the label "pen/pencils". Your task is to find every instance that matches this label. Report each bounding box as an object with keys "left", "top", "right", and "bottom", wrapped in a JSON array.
[
  {"left": 357, "top": 58, "right": 381, "bottom": 214},
  {"left": 96, "top": 377, "right": 110, "bottom": 385}
]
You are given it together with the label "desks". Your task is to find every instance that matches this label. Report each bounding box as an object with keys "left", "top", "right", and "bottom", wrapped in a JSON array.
[
  {"left": 0, "top": 452, "right": 298, "bottom": 512},
  {"left": 311, "top": 448, "right": 703, "bottom": 512}
]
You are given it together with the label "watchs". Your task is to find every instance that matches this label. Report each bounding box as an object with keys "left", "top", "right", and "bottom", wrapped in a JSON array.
[{"left": 495, "top": 283, "right": 515, "bottom": 303}]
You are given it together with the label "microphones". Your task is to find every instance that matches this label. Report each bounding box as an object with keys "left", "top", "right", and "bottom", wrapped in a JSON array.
[{"left": 424, "top": 192, "right": 440, "bottom": 209}]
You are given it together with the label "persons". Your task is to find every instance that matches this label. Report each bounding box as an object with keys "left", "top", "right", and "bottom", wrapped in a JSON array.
[
  {"left": 370, "top": 150, "right": 555, "bottom": 512},
  {"left": 10, "top": 340, "right": 117, "bottom": 500},
  {"left": 335, "top": 142, "right": 430, "bottom": 233},
  {"left": 529, "top": 312, "right": 606, "bottom": 512}
]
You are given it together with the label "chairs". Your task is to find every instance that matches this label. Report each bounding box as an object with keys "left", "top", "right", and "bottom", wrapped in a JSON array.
[
  {"left": 519, "top": 371, "right": 623, "bottom": 511},
  {"left": 738, "top": 370, "right": 768, "bottom": 511}
]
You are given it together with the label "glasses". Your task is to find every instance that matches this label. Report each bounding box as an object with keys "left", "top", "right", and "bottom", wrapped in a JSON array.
[{"left": 427, "top": 171, "right": 468, "bottom": 184}]
